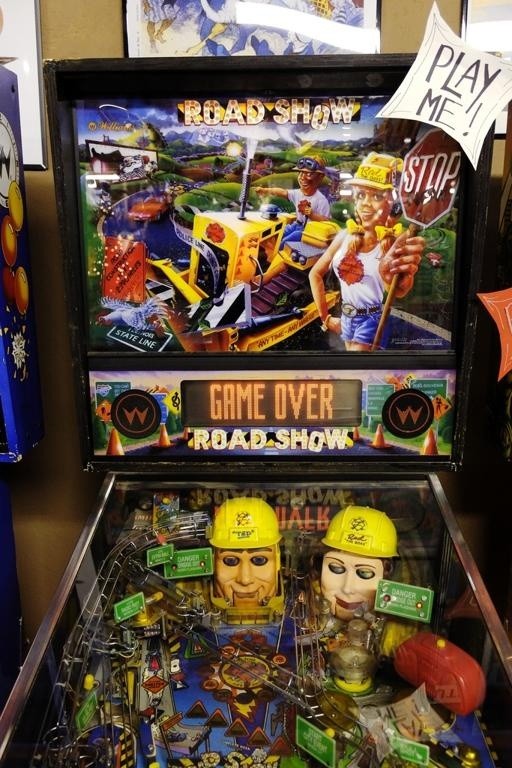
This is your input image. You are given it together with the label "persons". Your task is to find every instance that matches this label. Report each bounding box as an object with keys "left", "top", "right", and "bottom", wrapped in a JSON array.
[
  {"left": 305, "top": 152, "right": 425, "bottom": 350},
  {"left": 321, "top": 505, "right": 399, "bottom": 621},
  {"left": 250, "top": 154, "right": 332, "bottom": 288},
  {"left": 205, "top": 497, "right": 285, "bottom": 626}
]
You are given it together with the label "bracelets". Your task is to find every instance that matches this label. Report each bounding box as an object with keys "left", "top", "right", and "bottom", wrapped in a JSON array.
[{"left": 321, "top": 313, "right": 331, "bottom": 332}]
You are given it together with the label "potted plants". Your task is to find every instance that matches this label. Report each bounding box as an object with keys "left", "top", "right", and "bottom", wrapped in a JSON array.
[{"left": 0, "top": 0, "right": 48, "bottom": 170}]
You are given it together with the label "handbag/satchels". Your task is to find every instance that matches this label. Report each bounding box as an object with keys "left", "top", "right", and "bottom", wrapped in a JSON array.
[{"left": 321, "top": 313, "right": 331, "bottom": 332}]
